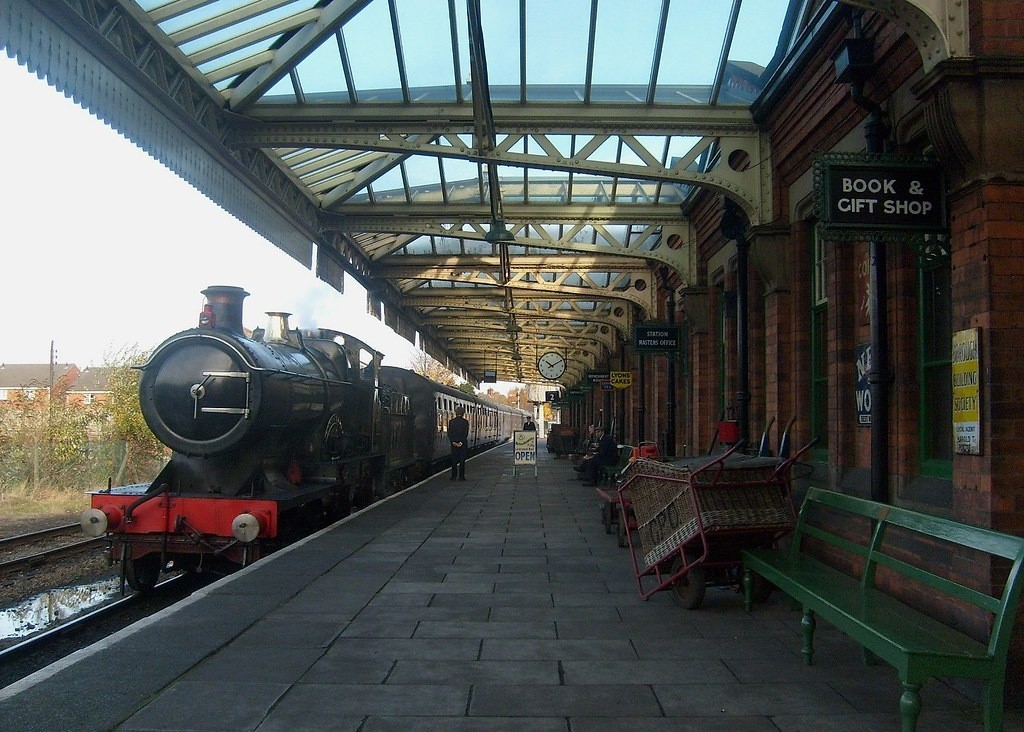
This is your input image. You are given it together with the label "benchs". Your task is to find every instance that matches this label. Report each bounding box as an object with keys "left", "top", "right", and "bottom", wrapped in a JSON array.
[
  {"left": 598, "top": 446, "right": 634, "bottom": 489},
  {"left": 739, "top": 485, "right": 1024, "bottom": 732}
]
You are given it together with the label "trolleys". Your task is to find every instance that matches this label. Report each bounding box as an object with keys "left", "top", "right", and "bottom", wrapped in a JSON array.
[{"left": 596, "top": 416, "right": 824, "bottom": 610}]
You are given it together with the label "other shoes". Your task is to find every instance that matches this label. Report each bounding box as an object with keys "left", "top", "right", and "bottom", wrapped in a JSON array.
[
  {"left": 449, "top": 476, "right": 457, "bottom": 481},
  {"left": 584, "top": 482, "right": 598, "bottom": 486},
  {"left": 459, "top": 477, "right": 466, "bottom": 481}
]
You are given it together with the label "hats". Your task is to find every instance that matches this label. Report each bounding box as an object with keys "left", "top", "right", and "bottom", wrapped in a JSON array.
[
  {"left": 456, "top": 407, "right": 464, "bottom": 416},
  {"left": 526, "top": 416, "right": 533, "bottom": 419},
  {"left": 594, "top": 426, "right": 606, "bottom": 432}
]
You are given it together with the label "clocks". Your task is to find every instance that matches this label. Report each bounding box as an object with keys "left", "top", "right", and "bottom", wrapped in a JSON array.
[{"left": 538, "top": 351, "right": 566, "bottom": 380}]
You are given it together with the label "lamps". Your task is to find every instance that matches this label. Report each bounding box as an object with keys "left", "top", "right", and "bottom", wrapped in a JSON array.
[
  {"left": 485, "top": 214, "right": 518, "bottom": 244},
  {"left": 517, "top": 373, "right": 524, "bottom": 378},
  {"left": 511, "top": 351, "right": 522, "bottom": 361},
  {"left": 505, "top": 317, "right": 524, "bottom": 332}
]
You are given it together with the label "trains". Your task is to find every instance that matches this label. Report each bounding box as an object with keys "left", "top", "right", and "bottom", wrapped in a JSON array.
[{"left": 79, "top": 286, "right": 534, "bottom": 597}]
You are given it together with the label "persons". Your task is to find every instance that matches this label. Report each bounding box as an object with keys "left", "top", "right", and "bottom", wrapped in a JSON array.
[
  {"left": 573, "top": 424, "right": 618, "bottom": 485},
  {"left": 523, "top": 416, "right": 536, "bottom": 430},
  {"left": 447, "top": 406, "right": 470, "bottom": 481}
]
